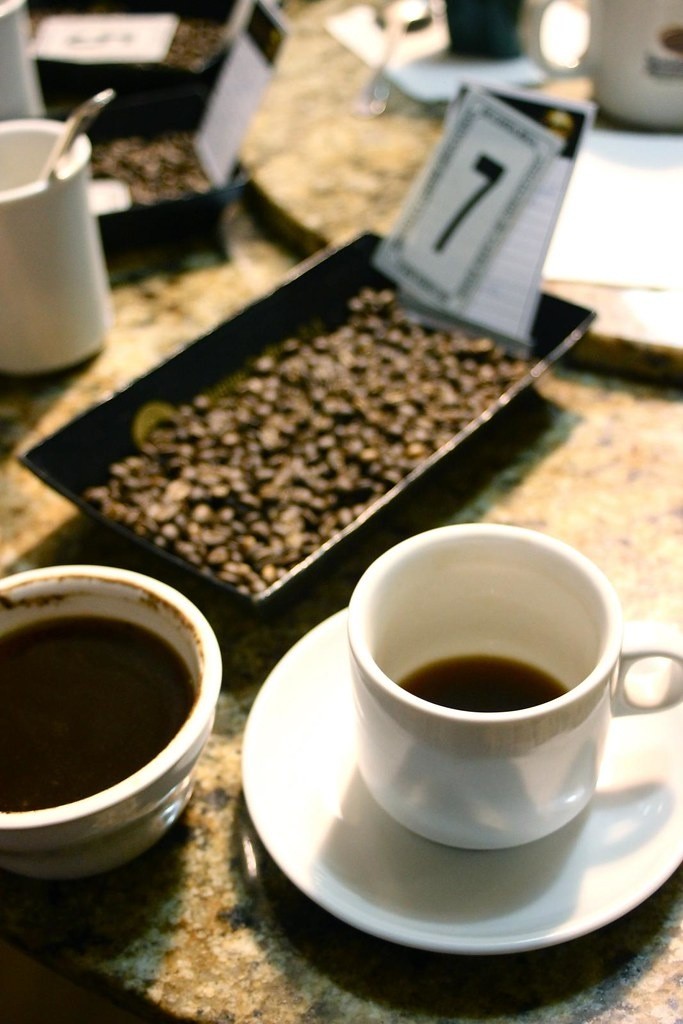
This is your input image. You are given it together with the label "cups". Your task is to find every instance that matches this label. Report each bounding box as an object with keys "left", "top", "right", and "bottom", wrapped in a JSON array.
[
  {"left": 0, "top": 121, "right": 115, "bottom": 375},
  {"left": 350, "top": 527, "right": 683, "bottom": 851},
  {"left": 446, "top": 0, "right": 524, "bottom": 58},
  {"left": 588, "top": 0, "right": 683, "bottom": 132},
  {"left": 0, "top": 0, "right": 48, "bottom": 120}
]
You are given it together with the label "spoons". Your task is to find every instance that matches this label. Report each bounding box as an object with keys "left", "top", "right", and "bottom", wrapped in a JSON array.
[{"left": 361, "top": 0, "right": 431, "bottom": 116}]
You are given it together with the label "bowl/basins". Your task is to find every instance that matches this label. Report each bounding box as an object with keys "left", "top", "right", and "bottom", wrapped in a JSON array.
[{"left": 0, "top": 565, "right": 223, "bottom": 881}]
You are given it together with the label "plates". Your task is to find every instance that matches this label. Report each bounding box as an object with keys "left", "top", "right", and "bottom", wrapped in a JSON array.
[
  {"left": 239, "top": 602, "right": 683, "bottom": 954},
  {"left": 62, "top": 103, "right": 249, "bottom": 244},
  {"left": 35, "top": 0, "right": 254, "bottom": 115},
  {"left": 325, "top": 3, "right": 542, "bottom": 100},
  {"left": 22, "top": 236, "right": 599, "bottom": 606}
]
